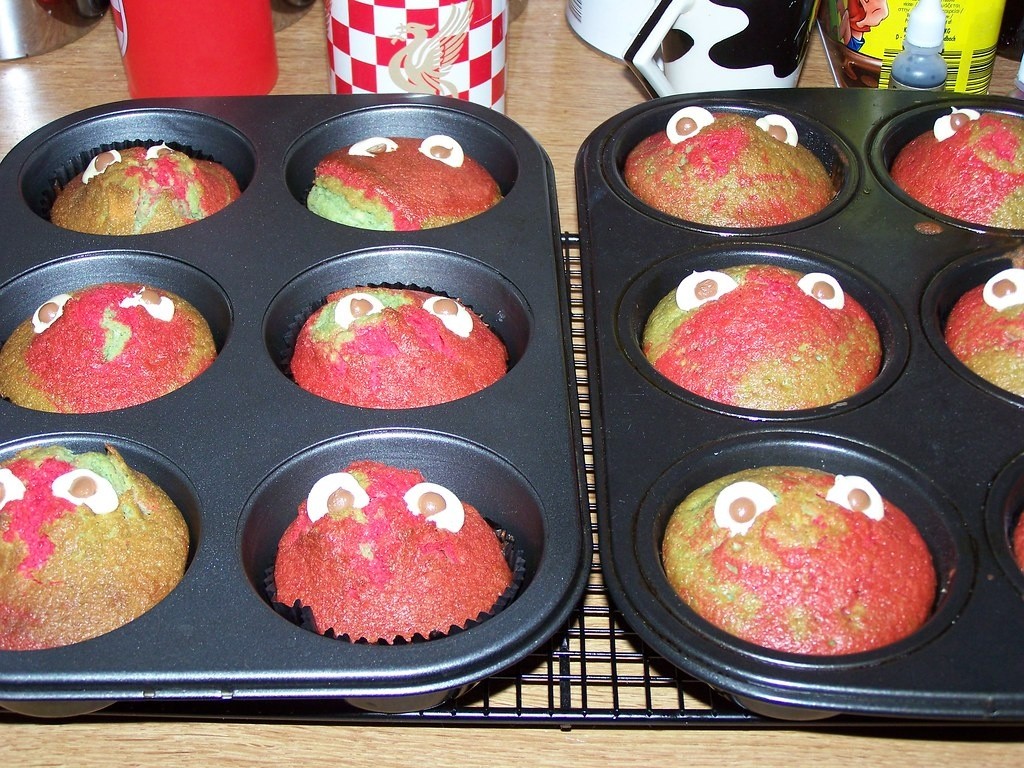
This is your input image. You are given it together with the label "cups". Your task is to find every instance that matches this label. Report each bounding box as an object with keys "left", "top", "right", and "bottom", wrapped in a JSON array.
[
  {"left": 323, "top": 0, "right": 508, "bottom": 115},
  {"left": 0, "top": 0, "right": 110, "bottom": 63},
  {"left": 111, "top": 0, "right": 279, "bottom": 99}
]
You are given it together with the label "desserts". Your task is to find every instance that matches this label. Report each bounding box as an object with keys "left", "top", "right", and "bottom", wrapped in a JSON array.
[
  {"left": 620, "top": 103, "right": 1024, "bottom": 653},
  {"left": 0, "top": 134, "right": 514, "bottom": 651}
]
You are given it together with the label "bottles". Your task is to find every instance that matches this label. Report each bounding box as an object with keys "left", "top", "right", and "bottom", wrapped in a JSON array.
[{"left": 887, "top": 0, "right": 948, "bottom": 91}]
